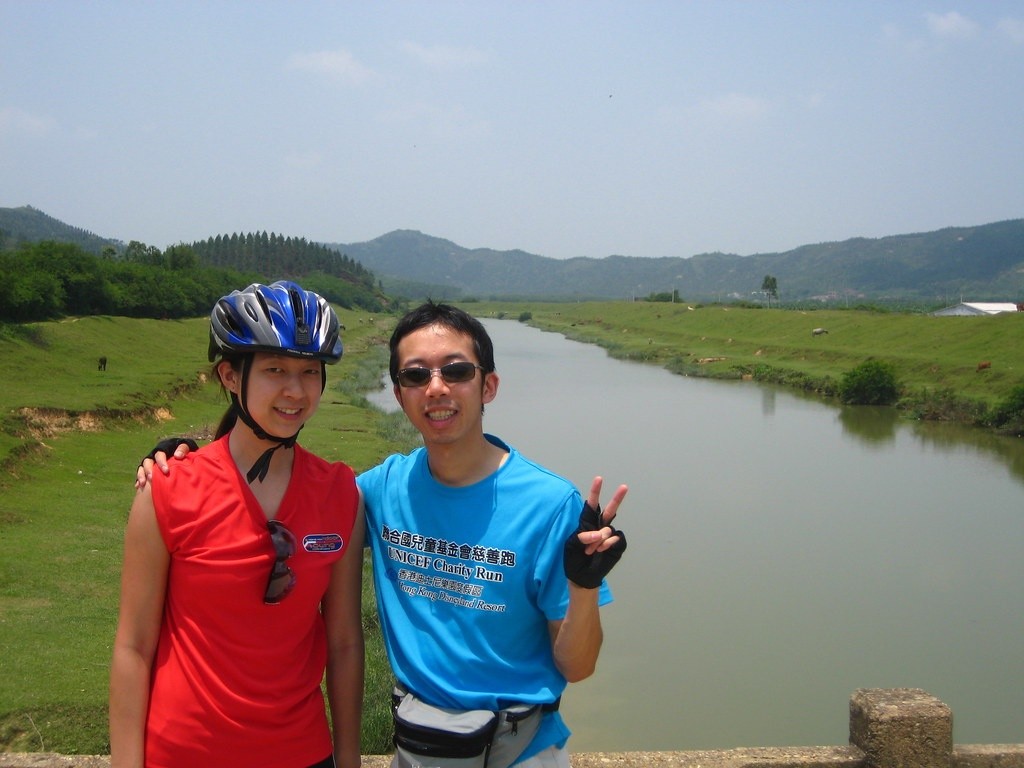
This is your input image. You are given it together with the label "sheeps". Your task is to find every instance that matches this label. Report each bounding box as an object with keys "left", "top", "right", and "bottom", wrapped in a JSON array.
[
  {"left": 812, "top": 328, "right": 829, "bottom": 337},
  {"left": 339, "top": 318, "right": 373, "bottom": 331}
]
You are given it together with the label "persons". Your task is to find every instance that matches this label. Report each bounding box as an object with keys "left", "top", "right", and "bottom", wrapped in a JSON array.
[
  {"left": 109, "top": 280, "right": 362, "bottom": 768},
  {"left": 135, "top": 302, "right": 627, "bottom": 768}
]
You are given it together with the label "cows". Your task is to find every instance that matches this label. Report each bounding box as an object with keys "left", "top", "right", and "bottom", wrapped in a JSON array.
[{"left": 96, "top": 355, "right": 107, "bottom": 371}]
